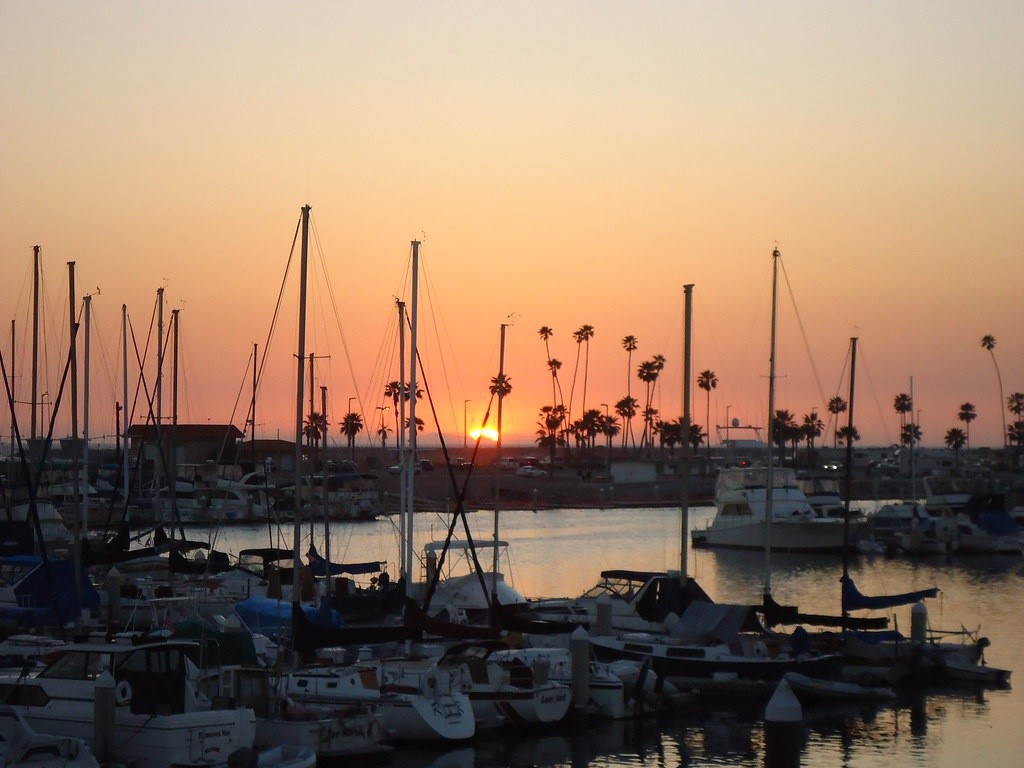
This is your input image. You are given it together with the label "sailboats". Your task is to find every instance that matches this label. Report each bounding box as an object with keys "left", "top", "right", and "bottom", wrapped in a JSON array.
[{"left": 1, "top": 204, "right": 1024, "bottom": 768}]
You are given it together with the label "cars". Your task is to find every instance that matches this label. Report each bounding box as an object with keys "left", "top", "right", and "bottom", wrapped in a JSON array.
[
  {"left": 522, "top": 455, "right": 540, "bottom": 467},
  {"left": 544, "top": 454, "right": 566, "bottom": 469},
  {"left": 502, "top": 457, "right": 521, "bottom": 471},
  {"left": 822, "top": 460, "right": 842, "bottom": 470},
  {"left": 454, "top": 457, "right": 475, "bottom": 468},
  {"left": 388, "top": 462, "right": 421, "bottom": 473}
]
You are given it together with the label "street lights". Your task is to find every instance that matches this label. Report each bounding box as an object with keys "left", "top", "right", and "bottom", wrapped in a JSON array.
[
  {"left": 601, "top": 403, "right": 609, "bottom": 446},
  {"left": 725, "top": 406, "right": 733, "bottom": 448},
  {"left": 41, "top": 393, "right": 49, "bottom": 439},
  {"left": 464, "top": 399, "right": 473, "bottom": 447},
  {"left": 348, "top": 396, "right": 357, "bottom": 415}
]
